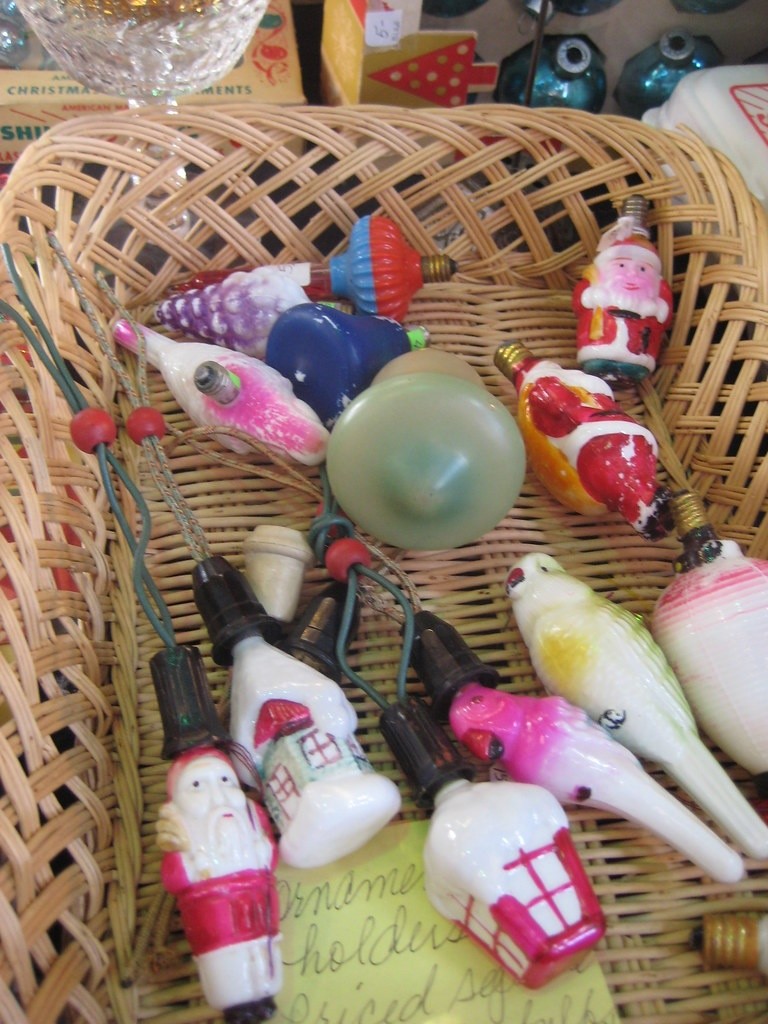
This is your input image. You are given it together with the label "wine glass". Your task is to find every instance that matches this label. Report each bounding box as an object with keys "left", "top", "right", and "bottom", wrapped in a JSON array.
[{"left": 12, "top": 0, "right": 273, "bottom": 276}]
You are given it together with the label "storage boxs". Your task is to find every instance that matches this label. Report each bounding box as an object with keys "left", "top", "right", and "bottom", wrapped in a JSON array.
[{"left": 0, "top": 0, "right": 306, "bottom": 165}]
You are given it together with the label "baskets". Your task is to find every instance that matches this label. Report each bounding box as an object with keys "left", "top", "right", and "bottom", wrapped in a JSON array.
[{"left": 0, "top": 104, "right": 768, "bottom": 1024}]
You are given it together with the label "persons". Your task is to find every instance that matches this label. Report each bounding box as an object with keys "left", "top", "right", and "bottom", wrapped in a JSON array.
[
  {"left": 154, "top": 744, "right": 280, "bottom": 1024},
  {"left": 516, "top": 356, "right": 674, "bottom": 543},
  {"left": 571, "top": 218, "right": 675, "bottom": 387}
]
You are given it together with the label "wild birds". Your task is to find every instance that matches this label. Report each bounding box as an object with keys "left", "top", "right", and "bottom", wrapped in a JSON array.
[
  {"left": 502, "top": 551, "right": 768, "bottom": 863},
  {"left": 447, "top": 679, "right": 748, "bottom": 887}
]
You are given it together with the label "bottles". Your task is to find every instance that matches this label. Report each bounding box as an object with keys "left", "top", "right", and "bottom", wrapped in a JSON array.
[{"left": 421, "top": 0, "right": 768, "bottom": 120}]
